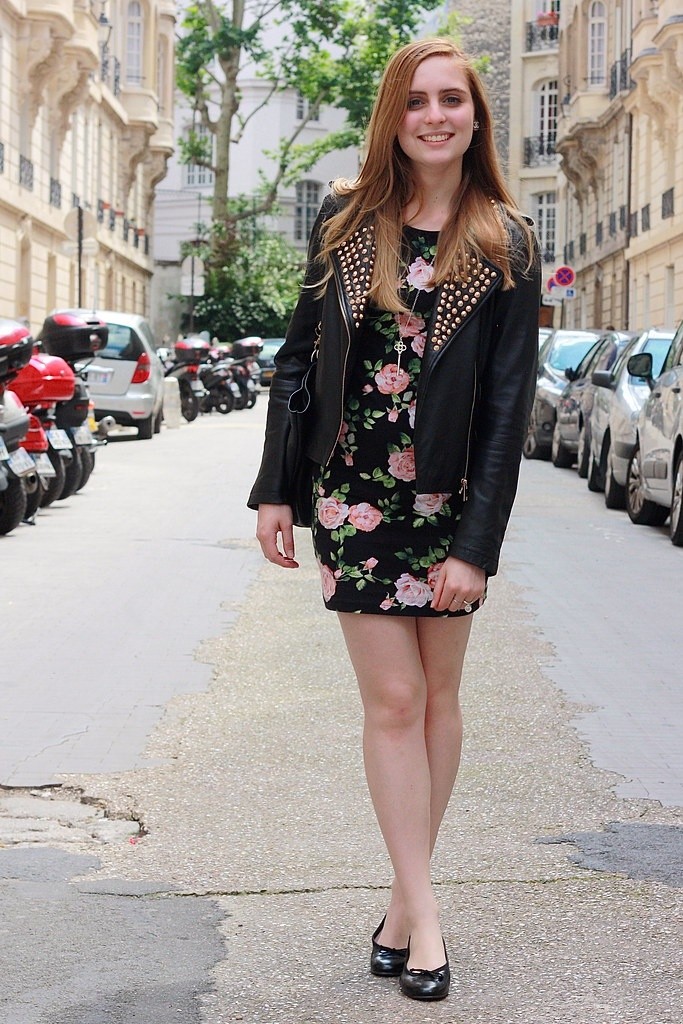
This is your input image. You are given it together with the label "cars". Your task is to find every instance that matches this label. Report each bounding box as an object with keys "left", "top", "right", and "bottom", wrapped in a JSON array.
[
  {"left": 257, "top": 338, "right": 289, "bottom": 385},
  {"left": 41, "top": 305, "right": 169, "bottom": 441},
  {"left": 520, "top": 294, "right": 683, "bottom": 548}
]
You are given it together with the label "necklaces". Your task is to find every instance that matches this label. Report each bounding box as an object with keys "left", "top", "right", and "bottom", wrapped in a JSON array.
[{"left": 394, "top": 224, "right": 438, "bottom": 376}]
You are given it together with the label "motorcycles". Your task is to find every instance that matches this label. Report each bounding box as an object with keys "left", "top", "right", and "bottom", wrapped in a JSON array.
[
  {"left": 0, "top": 306, "right": 114, "bottom": 537},
  {"left": 160, "top": 331, "right": 265, "bottom": 422}
]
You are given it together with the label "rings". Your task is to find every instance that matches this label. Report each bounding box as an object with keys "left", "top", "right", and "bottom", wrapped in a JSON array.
[
  {"left": 463, "top": 601, "right": 475, "bottom": 613},
  {"left": 453, "top": 598, "right": 463, "bottom": 605}
]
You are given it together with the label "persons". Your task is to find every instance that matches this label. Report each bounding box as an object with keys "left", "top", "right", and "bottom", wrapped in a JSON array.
[{"left": 248, "top": 38, "right": 540, "bottom": 1002}]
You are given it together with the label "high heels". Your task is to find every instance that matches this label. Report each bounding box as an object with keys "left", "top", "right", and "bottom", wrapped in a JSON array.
[{"left": 370, "top": 911, "right": 450, "bottom": 1002}]
